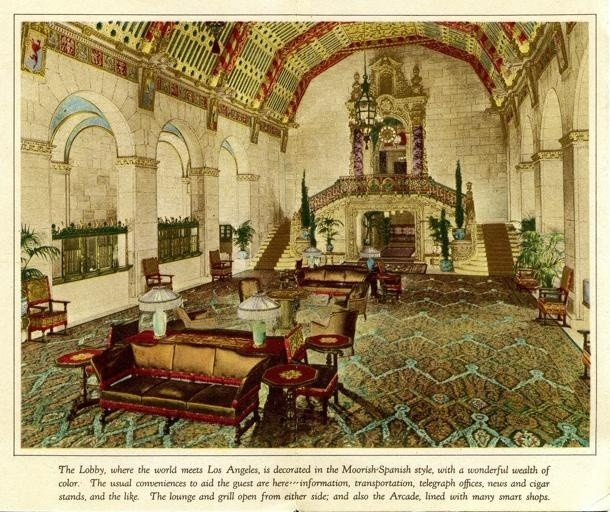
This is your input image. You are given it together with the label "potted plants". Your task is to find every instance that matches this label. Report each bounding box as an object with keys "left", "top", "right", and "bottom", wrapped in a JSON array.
[
  {"left": 299, "top": 169, "right": 345, "bottom": 253},
  {"left": 233, "top": 220, "right": 256, "bottom": 259},
  {"left": 418, "top": 159, "right": 468, "bottom": 271},
  {"left": 362, "top": 212, "right": 381, "bottom": 246}
]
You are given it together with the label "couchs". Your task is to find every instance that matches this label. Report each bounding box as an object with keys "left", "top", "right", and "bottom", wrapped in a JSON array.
[{"left": 91, "top": 343, "right": 270, "bottom": 446}]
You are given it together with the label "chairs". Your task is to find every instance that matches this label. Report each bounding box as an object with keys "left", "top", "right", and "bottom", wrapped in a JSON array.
[
  {"left": 209, "top": 249, "right": 234, "bottom": 282},
  {"left": 377, "top": 261, "right": 402, "bottom": 300},
  {"left": 338, "top": 283, "right": 368, "bottom": 319},
  {"left": 293, "top": 264, "right": 369, "bottom": 288},
  {"left": 240, "top": 278, "right": 264, "bottom": 302},
  {"left": 511, "top": 266, "right": 573, "bottom": 326},
  {"left": 142, "top": 257, "right": 174, "bottom": 293},
  {"left": 22, "top": 276, "right": 71, "bottom": 343}
]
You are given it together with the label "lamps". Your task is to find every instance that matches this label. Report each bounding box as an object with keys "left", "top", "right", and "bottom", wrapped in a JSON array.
[
  {"left": 354, "top": 22, "right": 377, "bottom": 151},
  {"left": 138, "top": 286, "right": 183, "bottom": 339},
  {"left": 302, "top": 249, "right": 321, "bottom": 267},
  {"left": 360, "top": 247, "right": 381, "bottom": 271},
  {"left": 236, "top": 292, "right": 281, "bottom": 348}
]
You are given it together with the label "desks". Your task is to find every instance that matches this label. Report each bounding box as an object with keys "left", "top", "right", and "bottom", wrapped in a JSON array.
[{"left": 117, "top": 330, "right": 284, "bottom": 354}]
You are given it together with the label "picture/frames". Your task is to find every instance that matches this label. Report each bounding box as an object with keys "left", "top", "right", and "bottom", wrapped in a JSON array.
[
  {"left": 139, "top": 68, "right": 155, "bottom": 113},
  {"left": 21, "top": 22, "right": 49, "bottom": 76},
  {"left": 550, "top": 25, "right": 572, "bottom": 77},
  {"left": 209, "top": 102, "right": 221, "bottom": 131},
  {"left": 525, "top": 73, "right": 539, "bottom": 109}
]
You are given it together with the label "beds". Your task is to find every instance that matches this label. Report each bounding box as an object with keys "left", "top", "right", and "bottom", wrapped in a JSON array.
[{"left": 55, "top": 349, "right": 102, "bottom": 415}]
